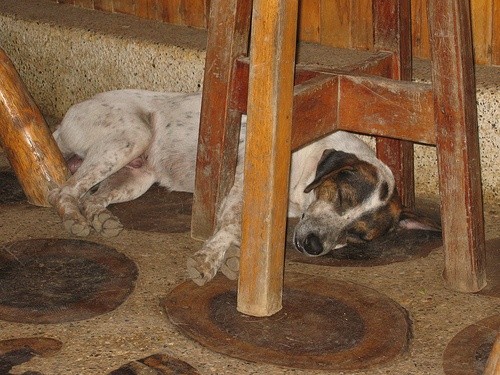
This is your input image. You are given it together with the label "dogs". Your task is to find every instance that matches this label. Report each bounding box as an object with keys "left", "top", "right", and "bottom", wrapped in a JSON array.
[{"left": 48, "top": 88, "right": 403, "bottom": 291}]
